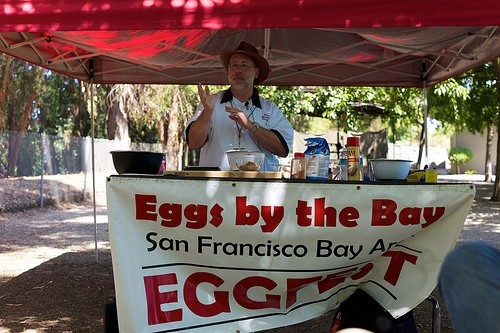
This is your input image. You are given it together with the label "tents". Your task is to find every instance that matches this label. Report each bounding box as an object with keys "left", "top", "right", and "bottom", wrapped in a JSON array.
[{"left": 1, "top": 0, "right": 500, "bottom": 263}]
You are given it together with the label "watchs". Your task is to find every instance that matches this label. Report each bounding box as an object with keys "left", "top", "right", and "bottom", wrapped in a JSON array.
[{"left": 249, "top": 122, "right": 260, "bottom": 133}]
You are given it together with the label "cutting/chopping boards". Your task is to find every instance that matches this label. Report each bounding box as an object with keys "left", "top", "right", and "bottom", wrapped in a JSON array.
[{"left": 174, "top": 170, "right": 282, "bottom": 179}]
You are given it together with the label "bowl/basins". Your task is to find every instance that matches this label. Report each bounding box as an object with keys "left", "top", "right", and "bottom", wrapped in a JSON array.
[
  {"left": 109, "top": 150, "right": 166, "bottom": 175},
  {"left": 225, "top": 150, "right": 265, "bottom": 172},
  {"left": 370, "top": 159, "right": 413, "bottom": 180}
]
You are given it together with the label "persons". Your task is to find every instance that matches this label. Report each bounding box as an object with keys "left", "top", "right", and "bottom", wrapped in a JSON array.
[{"left": 184, "top": 41, "right": 294, "bottom": 172}]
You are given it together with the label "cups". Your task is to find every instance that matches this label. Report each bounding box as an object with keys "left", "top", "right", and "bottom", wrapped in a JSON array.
[{"left": 425, "top": 169, "right": 437, "bottom": 183}]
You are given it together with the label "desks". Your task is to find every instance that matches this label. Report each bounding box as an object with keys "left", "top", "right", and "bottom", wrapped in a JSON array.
[{"left": 106, "top": 174, "right": 477, "bottom": 333}]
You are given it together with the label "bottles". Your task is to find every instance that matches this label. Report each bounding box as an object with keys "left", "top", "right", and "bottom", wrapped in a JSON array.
[
  {"left": 290, "top": 152, "right": 306, "bottom": 179},
  {"left": 331, "top": 160, "right": 341, "bottom": 180},
  {"left": 339, "top": 148, "right": 348, "bottom": 181},
  {"left": 346, "top": 137, "right": 360, "bottom": 181}
]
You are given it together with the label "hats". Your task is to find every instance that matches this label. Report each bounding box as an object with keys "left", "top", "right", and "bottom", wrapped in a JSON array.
[{"left": 219, "top": 41, "right": 270, "bottom": 85}]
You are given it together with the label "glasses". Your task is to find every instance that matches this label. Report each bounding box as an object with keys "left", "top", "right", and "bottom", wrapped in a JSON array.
[{"left": 228, "top": 62, "right": 256, "bottom": 70}]
